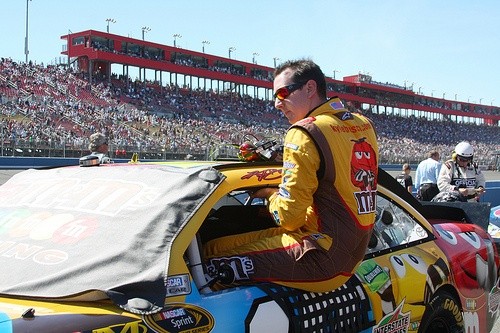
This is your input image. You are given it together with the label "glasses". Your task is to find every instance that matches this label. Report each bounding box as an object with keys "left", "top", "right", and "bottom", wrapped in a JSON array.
[{"left": 273, "top": 82, "right": 304, "bottom": 101}]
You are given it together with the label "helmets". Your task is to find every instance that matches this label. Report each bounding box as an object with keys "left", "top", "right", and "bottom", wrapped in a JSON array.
[{"left": 454, "top": 142, "right": 474, "bottom": 158}]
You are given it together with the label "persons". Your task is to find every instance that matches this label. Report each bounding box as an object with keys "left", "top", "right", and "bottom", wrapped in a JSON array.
[
  {"left": 0, "top": 29, "right": 499, "bottom": 172},
  {"left": 395, "top": 162, "right": 413, "bottom": 197},
  {"left": 436, "top": 140, "right": 485, "bottom": 208},
  {"left": 415, "top": 150, "right": 444, "bottom": 202},
  {"left": 195, "top": 59, "right": 379, "bottom": 293}
]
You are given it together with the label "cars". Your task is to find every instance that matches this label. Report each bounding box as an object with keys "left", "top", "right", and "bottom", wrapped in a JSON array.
[{"left": 1, "top": 160, "right": 500, "bottom": 333}]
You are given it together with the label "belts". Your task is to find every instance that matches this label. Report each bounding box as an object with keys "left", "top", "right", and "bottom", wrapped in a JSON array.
[{"left": 423, "top": 184, "right": 436, "bottom": 187}]
